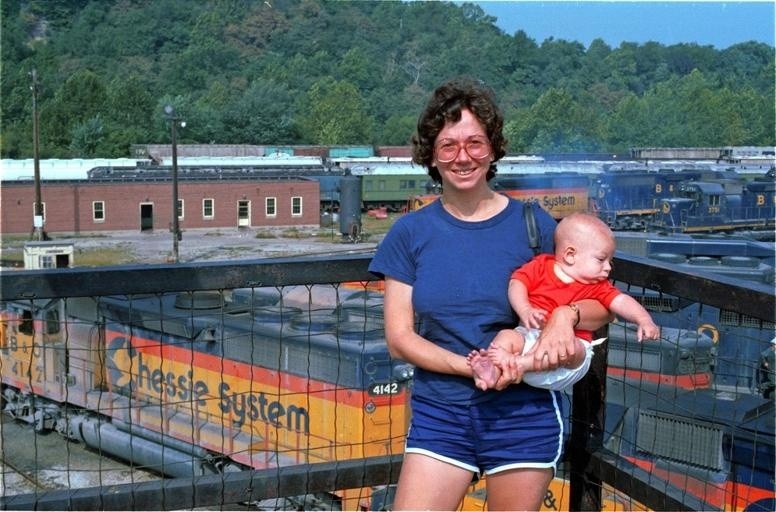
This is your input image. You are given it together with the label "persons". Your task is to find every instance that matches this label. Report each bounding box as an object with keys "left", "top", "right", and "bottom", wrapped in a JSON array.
[
  {"left": 366, "top": 81, "right": 615, "bottom": 511},
  {"left": 466, "top": 212, "right": 661, "bottom": 389}
]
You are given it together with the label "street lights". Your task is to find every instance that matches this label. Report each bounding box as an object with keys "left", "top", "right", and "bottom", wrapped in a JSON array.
[
  {"left": 163, "top": 104, "right": 187, "bottom": 265},
  {"left": 31, "top": 64, "right": 43, "bottom": 240}
]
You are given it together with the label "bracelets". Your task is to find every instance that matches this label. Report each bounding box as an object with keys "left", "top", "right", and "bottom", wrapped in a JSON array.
[{"left": 567, "top": 304, "right": 580, "bottom": 328}]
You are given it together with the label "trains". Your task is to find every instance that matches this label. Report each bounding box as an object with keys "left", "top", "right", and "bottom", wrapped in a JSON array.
[
  {"left": 0, "top": 279, "right": 776, "bottom": 511},
  {"left": 0, "top": 156, "right": 776, "bottom": 233}
]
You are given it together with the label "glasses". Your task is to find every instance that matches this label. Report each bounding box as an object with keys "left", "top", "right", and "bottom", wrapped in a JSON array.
[{"left": 433, "top": 135, "right": 493, "bottom": 163}]
